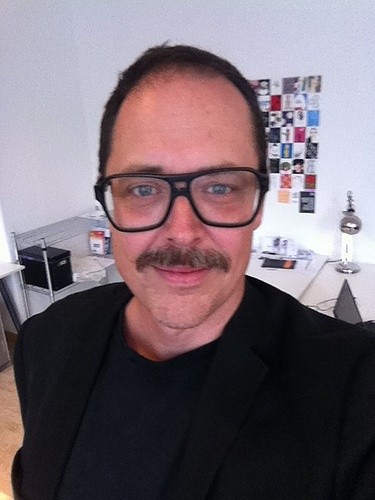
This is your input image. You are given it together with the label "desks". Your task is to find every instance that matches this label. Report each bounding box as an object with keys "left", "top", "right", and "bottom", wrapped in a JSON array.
[
  {"left": 244, "top": 250, "right": 375, "bottom": 325},
  {"left": 0, "top": 262, "right": 26, "bottom": 371}
]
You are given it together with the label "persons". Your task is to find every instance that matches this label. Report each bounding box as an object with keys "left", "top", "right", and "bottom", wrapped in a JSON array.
[
  {"left": 306, "top": 128, "right": 318, "bottom": 143},
  {"left": 11, "top": 40, "right": 375, "bottom": 500},
  {"left": 292, "top": 159, "right": 305, "bottom": 174},
  {"left": 307, "top": 76, "right": 320, "bottom": 92}
]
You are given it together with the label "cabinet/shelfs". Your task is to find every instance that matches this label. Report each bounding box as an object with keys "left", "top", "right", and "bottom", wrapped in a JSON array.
[{"left": 9, "top": 217, "right": 109, "bottom": 318}]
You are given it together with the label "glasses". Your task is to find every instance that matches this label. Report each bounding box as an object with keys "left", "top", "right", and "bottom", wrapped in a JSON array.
[{"left": 94, "top": 167, "right": 270, "bottom": 233}]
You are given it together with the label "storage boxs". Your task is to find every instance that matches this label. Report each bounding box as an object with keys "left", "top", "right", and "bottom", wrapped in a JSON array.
[
  {"left": 90, "top": 228, "right": 112, "bottom": 256},
  {"left": 18, "top": 246, "right": 73, "bottom": 292}
]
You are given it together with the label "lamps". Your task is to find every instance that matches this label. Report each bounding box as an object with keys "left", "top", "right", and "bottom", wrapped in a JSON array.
[{"left": 336, "top": 191, "right": 363, "bottom": 273}]
